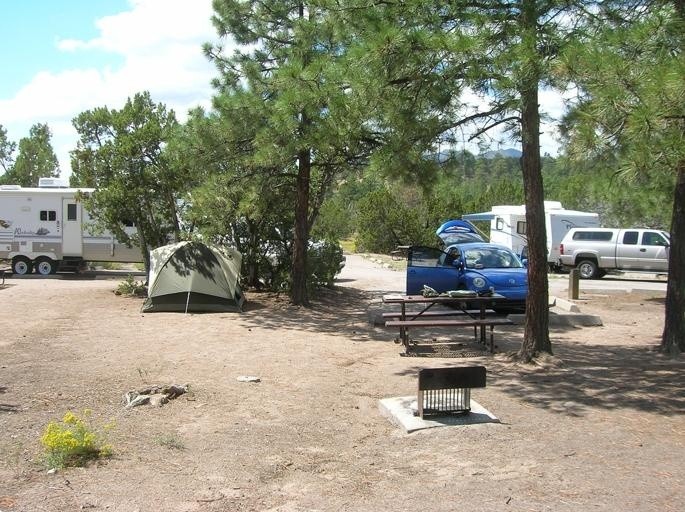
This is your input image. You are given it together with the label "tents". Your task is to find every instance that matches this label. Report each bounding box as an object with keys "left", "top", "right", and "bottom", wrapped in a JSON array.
[{"left": 141, "top": 239, "right": 247, "bottom": 313}]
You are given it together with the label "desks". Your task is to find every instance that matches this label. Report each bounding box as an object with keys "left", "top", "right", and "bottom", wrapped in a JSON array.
[{"left": 383, "top": 292, "right": 507, "bottom": 355}]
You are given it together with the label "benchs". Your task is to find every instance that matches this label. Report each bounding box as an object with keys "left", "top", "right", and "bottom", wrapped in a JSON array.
[
  {"left": 385, "top": 321, "right": 514, "bottom": 357},
  {"left": 382, "top": 311, "right": 498, "bottom": 346}
]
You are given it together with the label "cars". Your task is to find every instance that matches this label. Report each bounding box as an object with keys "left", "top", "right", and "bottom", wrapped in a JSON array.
[
  {"left": 559, "top": 227, "right": 670, "bottom": 280},
  {"left": 229, "top": 218, "right": 346, "bottom": 290},
  {"left": 406, "top": 220, "right": 528, "bottom": 309}
]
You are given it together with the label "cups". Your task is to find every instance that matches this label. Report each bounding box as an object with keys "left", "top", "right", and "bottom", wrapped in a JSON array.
[{"left": 489, "top": 287, "right": 494, "bottom": 294}]
